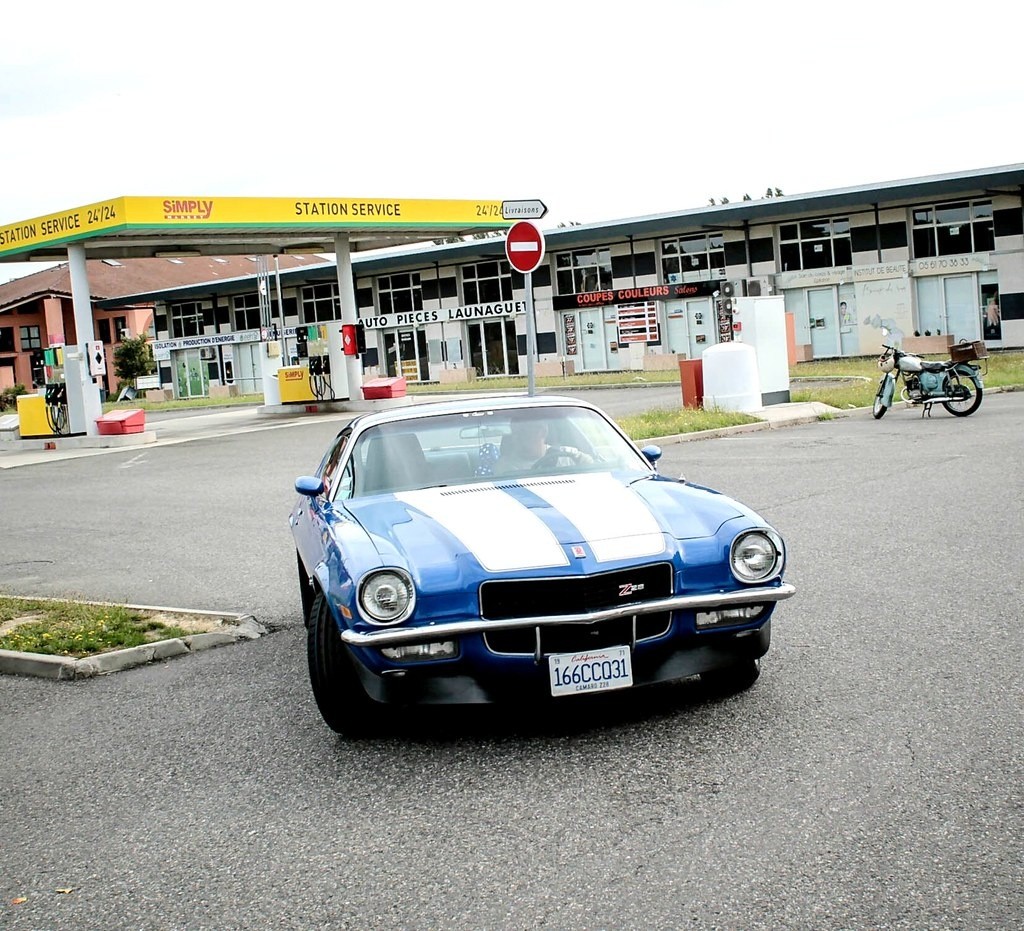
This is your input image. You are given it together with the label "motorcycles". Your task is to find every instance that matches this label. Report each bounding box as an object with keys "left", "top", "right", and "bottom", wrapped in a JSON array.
[{"left": 872, "top": 344, "right": 985, "bottom": 419}]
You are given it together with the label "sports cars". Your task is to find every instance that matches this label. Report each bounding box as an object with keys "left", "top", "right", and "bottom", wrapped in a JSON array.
[{"left": 289, "top": 394, "right": 796, "bottom": 738}]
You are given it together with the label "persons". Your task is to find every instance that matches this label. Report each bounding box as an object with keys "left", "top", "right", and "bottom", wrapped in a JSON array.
[{"left": 493, "top": 411, "right": 594, "bottom": 475}]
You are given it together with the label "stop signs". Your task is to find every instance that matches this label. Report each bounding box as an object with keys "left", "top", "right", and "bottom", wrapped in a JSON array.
[{"left": 504, "top": 221, "right": 545, "bottom": 274}]
